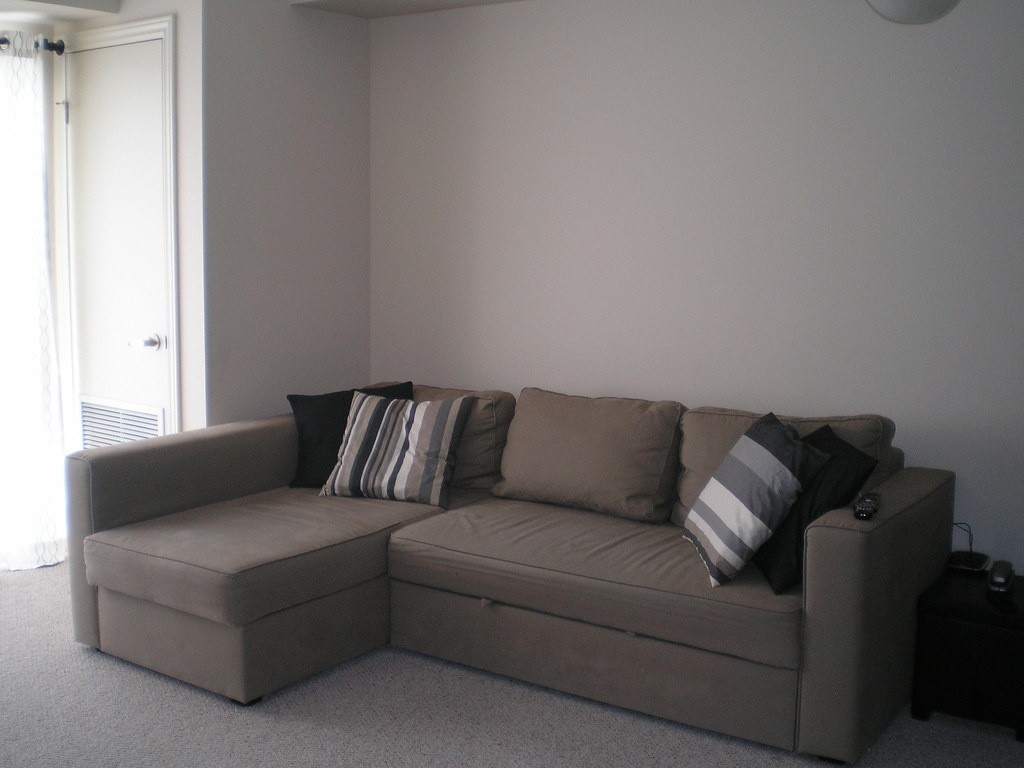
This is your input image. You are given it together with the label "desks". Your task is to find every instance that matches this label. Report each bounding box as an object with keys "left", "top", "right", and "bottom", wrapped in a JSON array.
[{"left": 911, "top": 563, "right": 1023, "bottom": 742}]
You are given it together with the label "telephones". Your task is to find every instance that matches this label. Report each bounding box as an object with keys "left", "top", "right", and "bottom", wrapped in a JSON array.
[{"left": 988, "top": 560, "right": 1016, "bottom": 595}]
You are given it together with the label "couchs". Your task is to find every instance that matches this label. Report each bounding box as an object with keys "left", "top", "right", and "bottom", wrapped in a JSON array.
[{"left": 65, "top": 414, "right": 959, "bottom": 768}]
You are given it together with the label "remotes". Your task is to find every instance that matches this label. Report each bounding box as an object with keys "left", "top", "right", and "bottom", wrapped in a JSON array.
[{"left": 854, "top": 493, "right": 881, "bottom": 520}]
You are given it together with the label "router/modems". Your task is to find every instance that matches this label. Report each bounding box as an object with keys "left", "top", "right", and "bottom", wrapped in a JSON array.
[{"left": 946, "top": 551, "right": 991, "bottom": 575}]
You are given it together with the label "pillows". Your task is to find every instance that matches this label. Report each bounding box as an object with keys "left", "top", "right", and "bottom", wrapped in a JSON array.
[
  {"left": 286, "top": 380, "right": 414, "bottom": 489},
  {"left": 319, "top": 390, "right": 473, "bottom": 508},
  {"left": 487, "top": 387, "right": 691, "bottom": 523},
  {"left": 413, "top": 386, "right": 516, "bottom": 489},
  {"left": 670, "top": 408, "right": 895, "bottom": 527},
  {"left": 683, "top": 411, "right": 831, "bottom": 588},
  {"left": 753, "top": 424, "right": 878, "bottom": 595}
]
